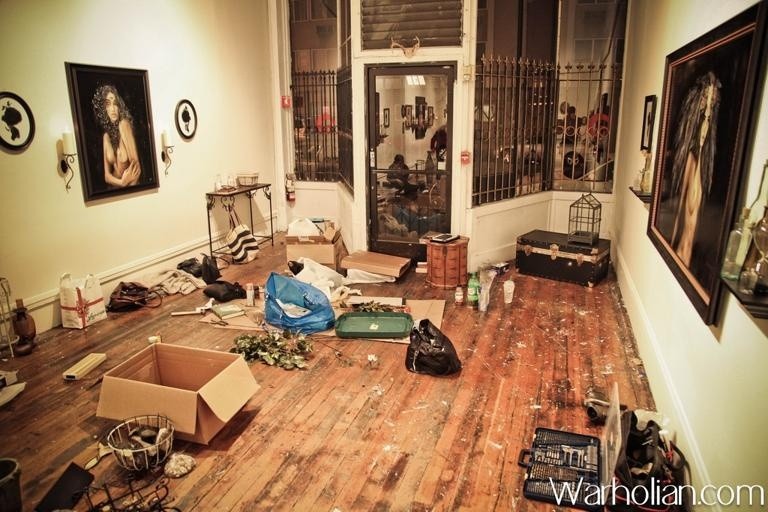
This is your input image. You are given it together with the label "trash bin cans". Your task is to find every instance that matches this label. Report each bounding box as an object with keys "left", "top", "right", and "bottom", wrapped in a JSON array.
[{"left": 2, "top": 459, "right": 22, "bottom": 511}]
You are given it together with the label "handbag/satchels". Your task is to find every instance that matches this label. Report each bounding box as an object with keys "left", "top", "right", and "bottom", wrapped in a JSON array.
[
  {"left": 59, "top": 272, "right": 107, "bottom": 329},
  {"left": 104, "top": 277, "right": 165, "bottom": 314},
  {"left": 404, "top": 317, "right": 463, "bottom": 377},
  {"left": 222, "top": 204, "right": 261, "bottom": 266},
  {"left": 202, "top": 276, "right": 247, "bottom": 303}
]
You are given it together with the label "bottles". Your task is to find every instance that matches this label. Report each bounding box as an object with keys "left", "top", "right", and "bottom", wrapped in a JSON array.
[
  {"left": 454, "top": 287, "right": 464, "bottom": 306},
  {"left": 467, "top": 271, "right": 482, "bottom": 310}
]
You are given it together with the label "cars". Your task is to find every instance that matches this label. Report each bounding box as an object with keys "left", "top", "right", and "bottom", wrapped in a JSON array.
[
  {"left": 431, "top": 121, "right": 515, "bottom": 161},
  {"left": 496, "top": 134, "right": 596, "bottom": 168}
]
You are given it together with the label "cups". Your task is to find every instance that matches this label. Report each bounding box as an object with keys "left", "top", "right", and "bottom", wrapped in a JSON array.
[{"left": 503, "top": 280, "right": 514, "bottom": 304}]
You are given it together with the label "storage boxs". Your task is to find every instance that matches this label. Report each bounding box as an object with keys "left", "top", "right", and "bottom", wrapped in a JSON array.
[
  {"left": 285, "top": 233, "right": 348, "bottom": 280},
  {"left": 340, "top": 247, "right": 410, "bottom": 277},
  {"left": 83, "top": 341, "right": 263, "bottom": 446}
]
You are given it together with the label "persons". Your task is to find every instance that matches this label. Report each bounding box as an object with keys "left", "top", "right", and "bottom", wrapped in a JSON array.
[
  {"left": 91, "top": 87, "right": 141, "bottom": 188},
  {"left": 415, "top": 114, "right": 425, "bottom": 140},
  {"left": 386, "top": 155, "right": 409, "bottom": 198},
  {"left": 589, "top": 93, "right": 610, "bottom": 163},
  {"left": 669, "top": 74, "right": 722, "bottom": 270},
  {"left": 430, "top": 123, "right": 447, "bottom": 177},
  {"left": 560, "top": 106, "right": 583, "bottom": 145}
]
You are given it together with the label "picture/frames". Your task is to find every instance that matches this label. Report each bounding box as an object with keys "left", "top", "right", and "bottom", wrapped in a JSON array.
[
  {"left": 64, "top": 58, "right": 162, "bottom": 207},
  {"left": 645, "top": 0, "right": 767, "bottom": 330},
  {"left": 639, "top": 96, "right": 659, "bottom": 153}
]
